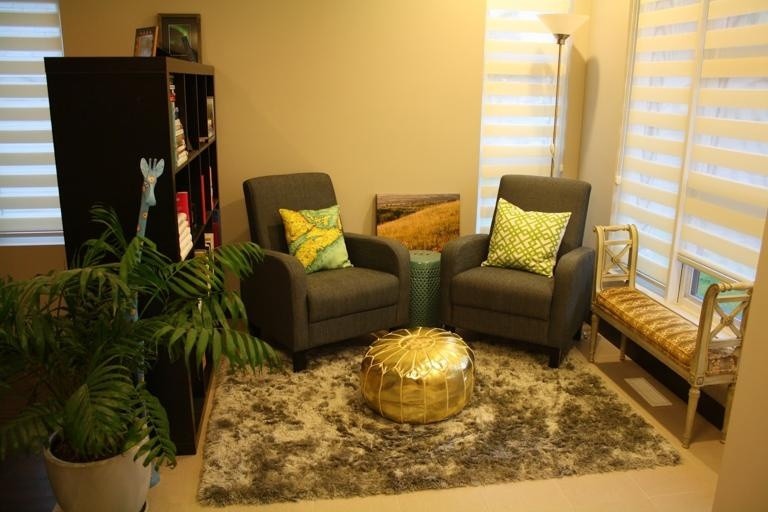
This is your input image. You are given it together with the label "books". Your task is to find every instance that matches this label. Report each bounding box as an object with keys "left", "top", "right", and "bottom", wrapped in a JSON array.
[{"left": 164, "top": 70, "right": 216, "bottom": 315}]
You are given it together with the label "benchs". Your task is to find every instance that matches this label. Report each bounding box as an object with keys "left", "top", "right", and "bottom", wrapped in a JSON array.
[{"left": 590, "top": 220, "right": 756, "bottom": 449}]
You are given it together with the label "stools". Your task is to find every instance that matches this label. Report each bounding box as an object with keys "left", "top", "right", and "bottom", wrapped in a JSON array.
[{"left": 361, "top": 326, "right": 477, "bottom": 426}]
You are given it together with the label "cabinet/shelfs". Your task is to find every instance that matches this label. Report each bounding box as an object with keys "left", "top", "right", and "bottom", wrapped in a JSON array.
[{"left": 41, "top": 50, "right": 226, "bottom": 456}]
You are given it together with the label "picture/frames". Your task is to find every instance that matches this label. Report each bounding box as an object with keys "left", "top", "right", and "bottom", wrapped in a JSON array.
[
  {"left": 158, "top": 11, "right": 204, "bottom": 67},
  {"left": 131, "top": 25, "right": 158, "bottom": 59}
]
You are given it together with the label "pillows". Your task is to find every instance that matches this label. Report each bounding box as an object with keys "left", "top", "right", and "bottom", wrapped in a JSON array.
[
  {"left": 481, "top": 196, "right": 573, "bottom": 277},
  {"left": 277, "top": 201, "right": 356, "bottom": 273}
]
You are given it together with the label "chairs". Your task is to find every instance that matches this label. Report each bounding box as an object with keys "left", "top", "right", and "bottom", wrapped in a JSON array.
[
  {"left": 240, "top": 169, "right": 405, "bottom": 377},
  {"left": 441, "top": 171, "right": 596, "bottom": 369}
]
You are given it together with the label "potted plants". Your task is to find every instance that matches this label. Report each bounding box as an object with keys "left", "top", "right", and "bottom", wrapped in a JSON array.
[{"left": 0, "top": 198, "right": 281, "bottom": 512}]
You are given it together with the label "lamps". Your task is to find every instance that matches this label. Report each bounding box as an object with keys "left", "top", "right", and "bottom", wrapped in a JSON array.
[{"left": 535, "top": 11, "right": 589, "bottom": 180}]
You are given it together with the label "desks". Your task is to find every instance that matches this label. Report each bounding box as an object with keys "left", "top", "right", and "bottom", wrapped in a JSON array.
[{"left": 407, "top": 249, "right": 444, "bottom": 331}]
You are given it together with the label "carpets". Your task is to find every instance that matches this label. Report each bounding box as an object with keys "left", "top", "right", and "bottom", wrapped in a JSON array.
[{"left": 191, "top": 306, "right": 682, "bottom": 510}]
